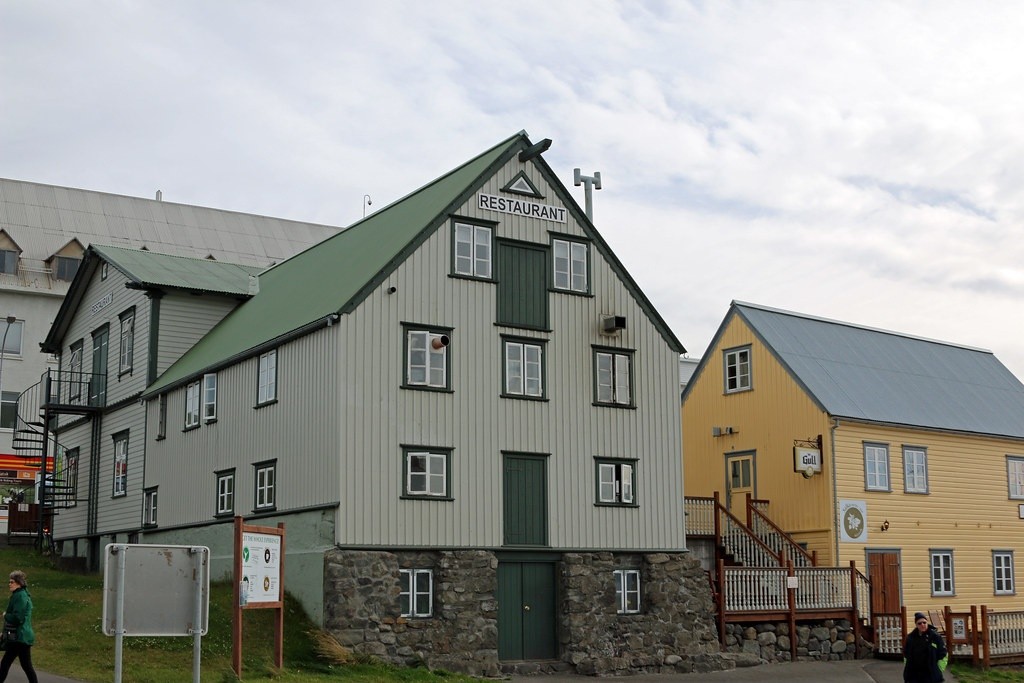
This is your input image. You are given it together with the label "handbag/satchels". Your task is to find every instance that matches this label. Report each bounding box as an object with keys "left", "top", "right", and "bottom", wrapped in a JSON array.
[{"left": 0, "top": 626, "right": 19, "bottom": 651}]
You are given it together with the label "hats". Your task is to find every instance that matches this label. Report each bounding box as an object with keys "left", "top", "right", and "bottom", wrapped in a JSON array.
[{"left": 914, "top": 613, "right": 927, "bottom": 622}]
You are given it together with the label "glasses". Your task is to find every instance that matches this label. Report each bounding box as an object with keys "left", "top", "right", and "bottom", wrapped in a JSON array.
[
  {"left": 918, "top": 622, "right": 927, "bottom": 626},
  {"left": 8, "top": 581, "right": 15, "bottom": 584}
]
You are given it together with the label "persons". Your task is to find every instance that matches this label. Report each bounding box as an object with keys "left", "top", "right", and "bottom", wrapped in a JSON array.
[
  {"left": 902, "top": 612, "right": 949, "bottom": 683},
  {"left": 0, "top": 570, "right": 38, "bottom": 683}
]
